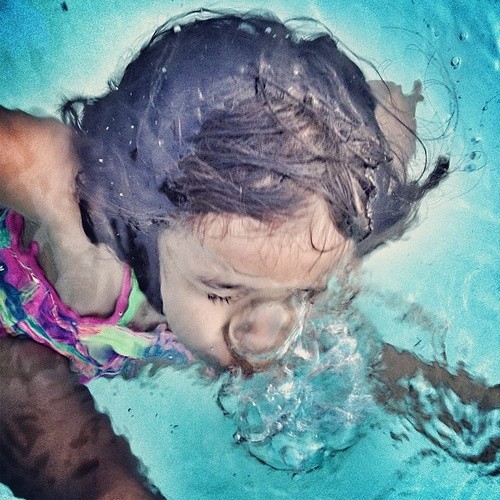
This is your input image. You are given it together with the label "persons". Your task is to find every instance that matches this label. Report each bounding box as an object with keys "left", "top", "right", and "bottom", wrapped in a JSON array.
[{"left": 1, "top": 1, "right": 499, "bottom": 500}]
